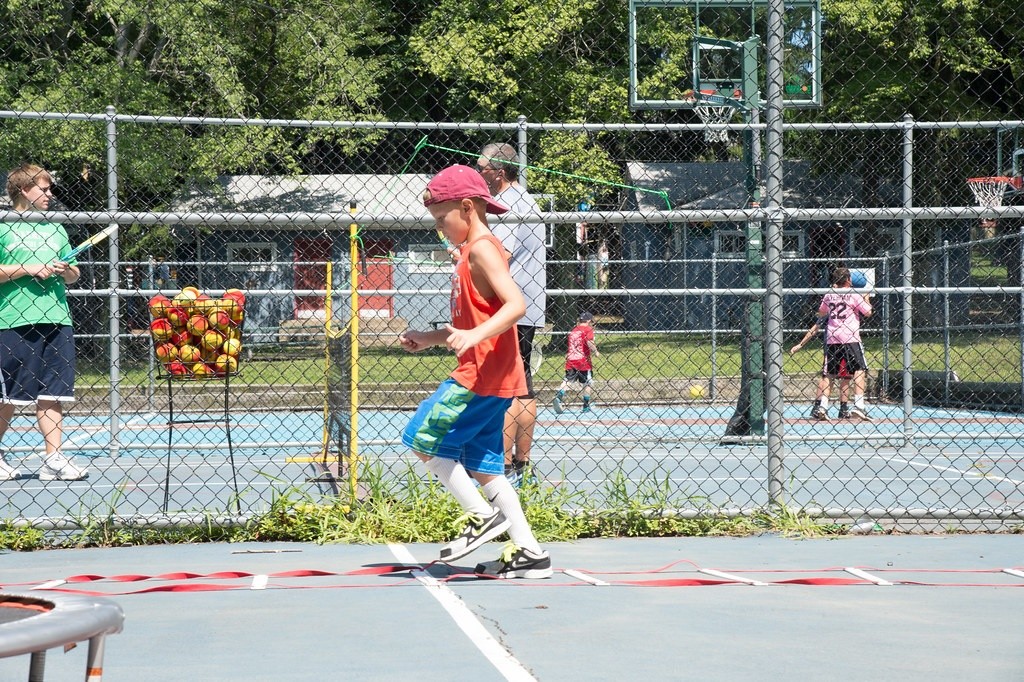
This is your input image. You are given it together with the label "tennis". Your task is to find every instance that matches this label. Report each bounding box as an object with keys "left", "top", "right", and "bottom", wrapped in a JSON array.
[{"left": 150, "top": 286, "right": 244, "bottom": 380}]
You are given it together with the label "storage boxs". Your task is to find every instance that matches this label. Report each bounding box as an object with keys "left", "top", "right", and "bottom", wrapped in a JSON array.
[{"left": 146, "top": 297, "right": 248, "bottom": 381}]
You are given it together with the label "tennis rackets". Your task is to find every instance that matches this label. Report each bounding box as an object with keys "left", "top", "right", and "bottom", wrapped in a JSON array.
[{"left": 34, "top": 224, "right": 119, "bottom": 282}]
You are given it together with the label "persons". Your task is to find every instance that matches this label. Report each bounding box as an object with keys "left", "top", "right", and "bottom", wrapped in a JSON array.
[
  {"left": 398, "top": 162, "right": 555, "bottom": 580},
  {"left": 157, "top": 255, "right": 170, "bottom": 290},
  {"left": 1, "top": 163, "right": 89, "bottom": 483},
  {"left": 791, "top": 268, "right": 876, "bottom": 423},
  {"left": 553, "top": 310, "right": 600, "bottom": 415},
  {"left": 475, "top": 144, "right": 549, "bottom": 499}
]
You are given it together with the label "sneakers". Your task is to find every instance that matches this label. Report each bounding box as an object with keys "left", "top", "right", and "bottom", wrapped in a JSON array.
[
  {"left": 583, "top": 405, "right": 591, "bottom": 412},
  {"left": 852, "top": 405, "right": 873, "bottom": 420},
  {"left": 39, "top": 457, "right": 89, "bottom": 481},
  {"left": 553, "top": 397, "right": 564, "bottom": 414},
  {"left": 814, "top": 406, "right": 832, "bottom": 420},
  {"left": 838, "top": 409, "right": 850, "bottom": 418},
  {"left": 441, "top": 506, "right": 511, "bottom": 563},
  {"left": 0, "top": 449, "right": 22, "bottom": 480},
  {"left": 505, "top": 466, "right": 538, "bottom": 494},
  {"left": 473, "top": 540, "right": 553, "bottom": 579}
]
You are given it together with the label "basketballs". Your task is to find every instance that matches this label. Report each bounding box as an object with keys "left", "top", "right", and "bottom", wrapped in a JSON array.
[
  {"left": 852, "top": 271, "right": 867, "bottom": 288},
  {"left": 690, "top": 385, "right": 704, "bottom": 399}
]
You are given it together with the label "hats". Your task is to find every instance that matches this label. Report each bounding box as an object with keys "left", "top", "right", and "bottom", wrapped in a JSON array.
[{"left": 424, "top": 164, "right": 509, "bottom": 214}]
